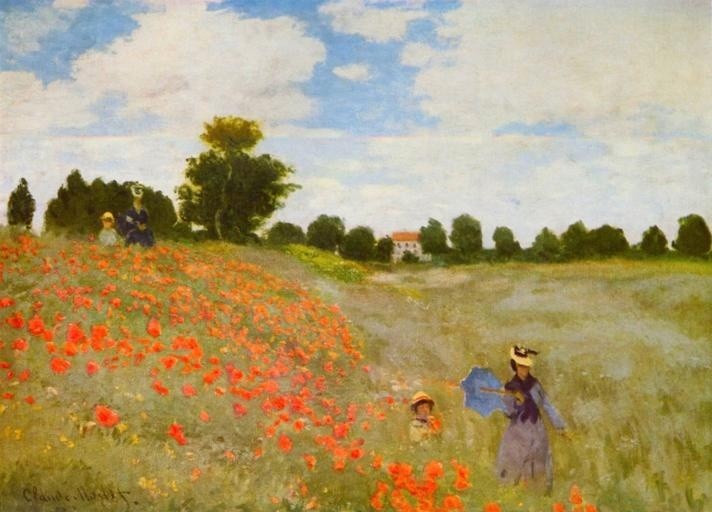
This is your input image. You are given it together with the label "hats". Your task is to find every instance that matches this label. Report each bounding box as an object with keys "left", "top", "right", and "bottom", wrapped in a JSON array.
[
  {"left": 510, "top": 345, "right": 533, "bottom": 366},
  {"left": 130, "top": 184, "right": 144, "bottom": 196},
  {"left": 411, "top": 392, "right": 435, "bottom": 410},
  {"left": 100, "top": 211, "right": 115, "bottom": 222}
]
